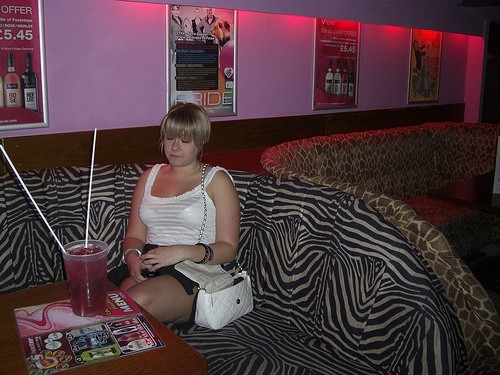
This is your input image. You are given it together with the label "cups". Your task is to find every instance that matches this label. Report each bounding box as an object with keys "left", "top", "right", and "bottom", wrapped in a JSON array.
[{"left": 61, "top": 240, "right": 108, "bottom": 317}]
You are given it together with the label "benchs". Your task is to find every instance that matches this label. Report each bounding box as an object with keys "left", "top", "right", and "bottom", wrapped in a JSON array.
[
  {"left": 0, "top": 165, "right": 500, "bottom": 375},
  {"left": 260, "top": 121, "right": 500, "bottom": 374}
]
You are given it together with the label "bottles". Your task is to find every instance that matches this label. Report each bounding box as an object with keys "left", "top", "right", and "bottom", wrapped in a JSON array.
[
  {"left": 0, "top": 52, "right": 39, "bottom": 112},
  {"left": 325, "top": 59, "right": 354, "bottom": 97}
]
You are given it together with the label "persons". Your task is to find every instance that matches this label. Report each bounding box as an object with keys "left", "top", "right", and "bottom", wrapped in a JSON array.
[{"left": 120, "top": 102, "right": 240, "bottom": 324}]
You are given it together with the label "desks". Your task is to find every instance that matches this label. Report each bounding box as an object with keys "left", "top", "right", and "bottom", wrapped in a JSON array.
[
  {"left": 1, "top": 275, "right": 209, "bottom": 375},
  {"left": 428, "top": 170, "right": 500, "bottom": 218}
]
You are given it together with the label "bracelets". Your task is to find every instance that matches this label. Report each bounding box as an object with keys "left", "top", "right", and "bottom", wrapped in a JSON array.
[
  {"left": 193, "top": 243, "right": 214, "bottom": 265},
  {"left": 122, "top": 247, "right": 141, "bottom": 265}
]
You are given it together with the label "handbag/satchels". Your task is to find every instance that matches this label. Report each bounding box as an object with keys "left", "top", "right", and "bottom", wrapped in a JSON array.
[{"left": 174, "top": 259, "right": 253, "bottom": 330}]
